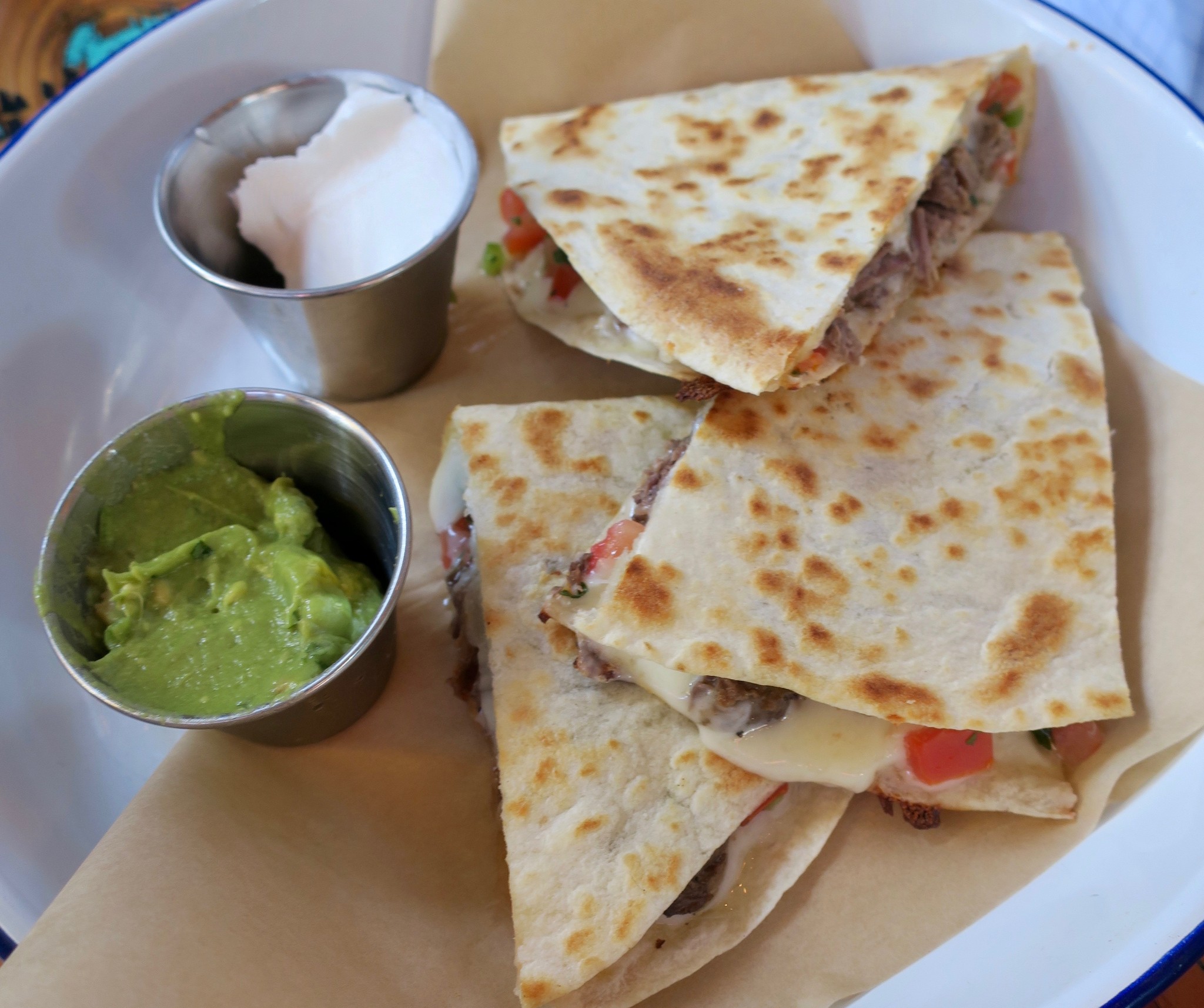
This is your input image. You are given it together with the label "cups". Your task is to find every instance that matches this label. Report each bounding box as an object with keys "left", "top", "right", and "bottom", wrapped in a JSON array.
[
  {"left": 151, "top": 67, "right": 480, "bottom": 402},
  {"left": 33, "top": 387, "right": 414, "bottom": 747}
]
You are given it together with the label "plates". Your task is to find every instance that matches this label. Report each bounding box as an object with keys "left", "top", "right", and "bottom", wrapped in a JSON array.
[{"left": 1, "top": 2, "right": 1204, "bottom": 1008}]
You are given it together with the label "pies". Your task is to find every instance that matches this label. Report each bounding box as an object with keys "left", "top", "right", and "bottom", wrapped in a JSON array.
[
  {"left": 428, "top": 396, "right": 855, "bottom": 1008},
  {"left": 486, "top": 43, "right": 1037, "bottom": 394},
  {"left": 538, "top": 231, "right": 1134, "bottom": 821}
]
